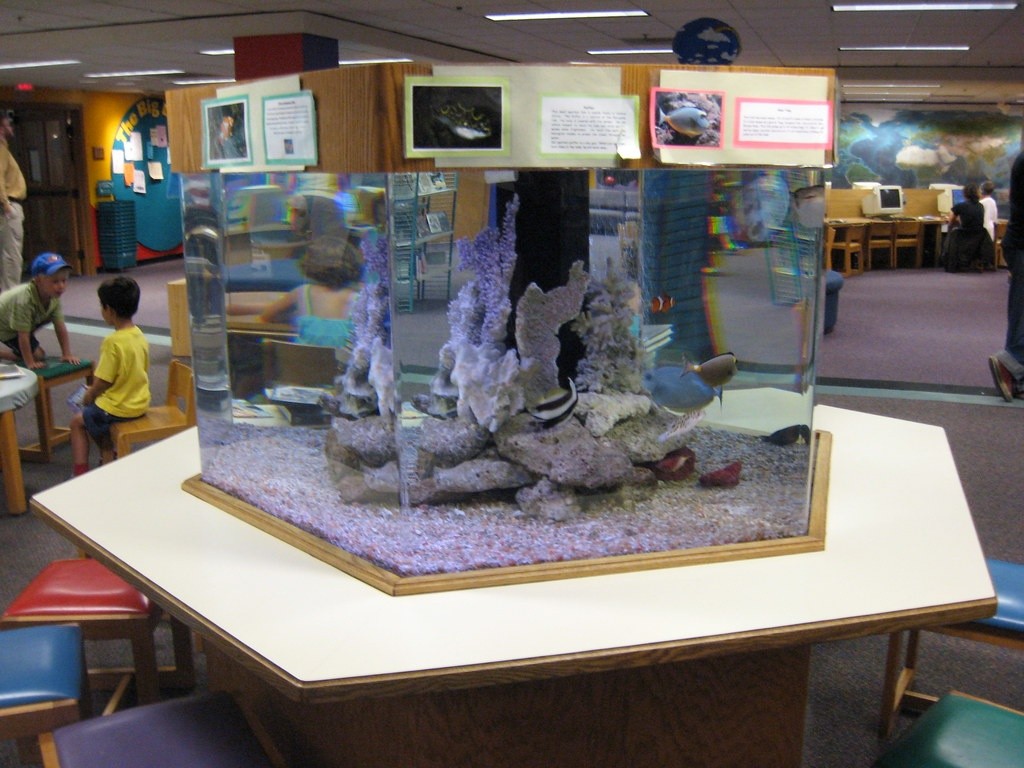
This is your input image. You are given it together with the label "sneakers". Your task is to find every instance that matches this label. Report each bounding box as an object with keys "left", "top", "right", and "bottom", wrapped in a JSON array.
[{"left": 989, "top": 355, "right": 1013, "bottom": 402}]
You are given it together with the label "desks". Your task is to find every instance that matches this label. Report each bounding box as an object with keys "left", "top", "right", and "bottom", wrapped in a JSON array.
[
  {"left": 226, "top": 313, "right": 296, "bottom": 379},
  {"left": 820, "top": 189, "right": 941, "bottom": 225},
  {"left": 0, "top": 363, "right": 39, "bottom": 515},
  {"left": 230, "top": 221, "right": 365, "bottom": 295},
  {"left": 31, "top": 384, "right": 998, "bottom": 767}
]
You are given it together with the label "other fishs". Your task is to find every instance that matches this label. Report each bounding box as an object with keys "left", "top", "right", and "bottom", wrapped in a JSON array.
[
  {"left": 656, "top": 409, "right": 706, "bottom": 441},
  {"left": 698, "top": 266, "right": 724, "bottom": 277},
  {"left": 725, "top": 175, "right": 788, "bottom": 240},
  {"left": 639, "top": 364, "right": 724, "bottom": 412},
  {"left": 434, "top": 102, "right": 491, "bottom": 134},
  {"left": 649, "top": 292, "right": 675, "bottom": 313},
  {"left": 680, "top": 351, "right": 740, "bottom": 385},
  {"left": 654, "top": 106, "right": 714, "bottom": 139},
  {"left": 525, "top": 374, "right": 577, "bottom": 424}
]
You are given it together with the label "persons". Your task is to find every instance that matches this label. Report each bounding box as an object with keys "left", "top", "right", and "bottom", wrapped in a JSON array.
[
  {"left": 978, "top": 179, "right": 998, "bottom": 242},
  {"left": 0, "top": 106, "right": 27, "bottom": 293},
  {"left": 0, "top": 251, "right": 81, "bottom": 370},
  {"left": 988, "top": 149, "right": 1024, "bottom": 404},
  {"left": 371, "top": 191, "right": 388, "bottom": 227},
  {"left": 260, "top": 235, "right": 364, "bottom": 349},
  {"left": 288, "top": 168, "right": 349, "bottom": 256},
  {"left": 947, "top": 182, "right": 995, "bottom": 272},
  {"left": 67, "top": 275, "right": 152, "bottom": 478}
]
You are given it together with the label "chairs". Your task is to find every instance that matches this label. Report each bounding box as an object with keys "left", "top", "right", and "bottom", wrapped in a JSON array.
[
  {"left": 824, "top": 220, "right": 1008, "bottom": 277},
  {"left": 262, "top": 340, "right": 336, "bottom": 395}
]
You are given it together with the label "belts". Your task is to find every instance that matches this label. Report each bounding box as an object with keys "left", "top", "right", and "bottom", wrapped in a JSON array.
[{"left": 8, "top": 196, "right": 22, "bottom": 204}]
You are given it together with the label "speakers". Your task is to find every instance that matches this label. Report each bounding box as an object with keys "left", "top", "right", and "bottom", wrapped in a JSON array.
[{"left": 63, "top": 109, "right": 81, "bottom": 140}]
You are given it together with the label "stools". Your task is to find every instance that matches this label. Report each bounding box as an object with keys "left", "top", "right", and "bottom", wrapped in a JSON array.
[
  {"left": 863, "top": 558, "right": 1024, "bottom": 768},
  {"left": 825, "top": 270, "right": 843, "bottom": 333},
  {"left": 0, "top": 362, "right": 282, "bottom": 768}
]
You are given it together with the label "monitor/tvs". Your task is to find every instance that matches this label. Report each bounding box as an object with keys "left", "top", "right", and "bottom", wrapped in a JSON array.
[
  {"left": 937, "top": 186, "right": 965, "bottom": 216},
  {"left": 862, "top": 186, "right": 904, "bottom": 217}
]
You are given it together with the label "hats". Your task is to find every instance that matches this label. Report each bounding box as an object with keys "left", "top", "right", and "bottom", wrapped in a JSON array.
[{"left": 32, "top": 253, "right": 72, "bottom": 276}]
